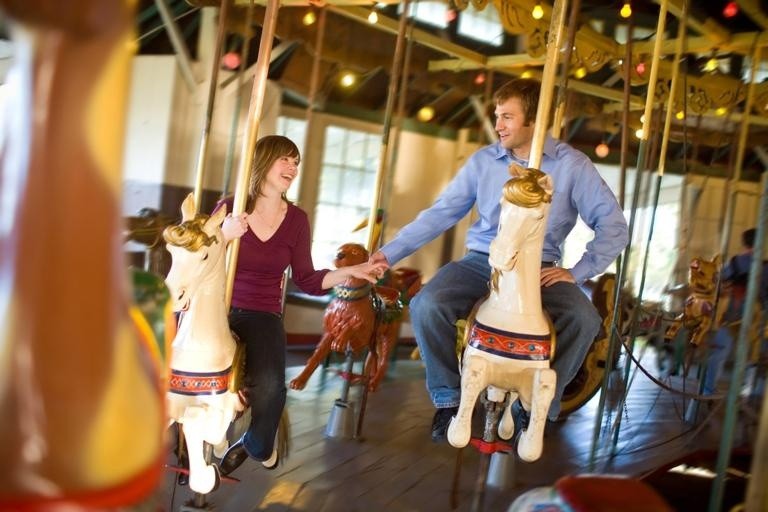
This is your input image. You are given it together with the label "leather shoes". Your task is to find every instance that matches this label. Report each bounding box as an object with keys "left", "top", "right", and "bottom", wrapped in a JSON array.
[
  {"left": 174, "top": 447, "right": 189, "bottom": 485},
  {"left": 218, "top": 441, "right": 249, "bottom": 475},
  {"left": 512, "top": 400, "right": 529, "bottom": 438},
  {"left": 431, "top": 406, "right": 457, "bottom": 441}
]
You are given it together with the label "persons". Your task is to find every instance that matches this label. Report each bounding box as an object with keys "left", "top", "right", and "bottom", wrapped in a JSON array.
[
  {"left": 179, "top": 135, "right": 389, "bottom": 486},
  {"left": 702, "top": 228, "right": 758, "bottom": 396},
  {"left": 369, "top": 78, "right": 628, "bottom": 442}
]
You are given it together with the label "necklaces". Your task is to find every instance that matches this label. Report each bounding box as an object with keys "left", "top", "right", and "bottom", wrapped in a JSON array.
[{"left": 255, "top": 206, "right": 283, "bottom": 229}]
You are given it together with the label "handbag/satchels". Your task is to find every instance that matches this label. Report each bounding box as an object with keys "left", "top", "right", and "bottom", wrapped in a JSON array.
[{"left": 720, "top": 255, "right": 749, "bottom": 328}]
